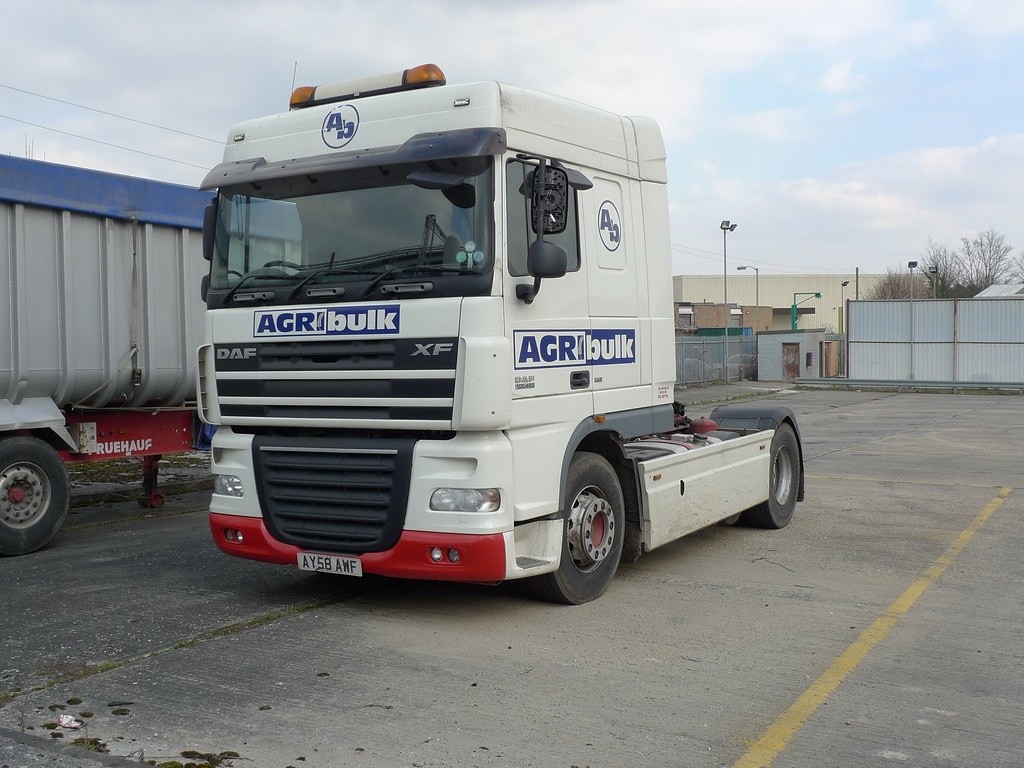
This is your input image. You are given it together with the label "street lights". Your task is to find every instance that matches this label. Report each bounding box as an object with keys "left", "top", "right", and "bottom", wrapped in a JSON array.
[
  {"left": 737, "top": 266, "right": 759, "bottom": 332},
  {"left": 908, "top": 262, "right": 917, "bottom": 380},
  {"left": 792, "top": 293, "right": 822, "bottom": 331},
  {"left": 929, "top": 266, "right": 937, "bottom": 299},
  {"left": 720, "top": 220, "right": 737, "bottom": 384},
  {"left": 841, "top": 280, "right": 849, "bottom": 334}
]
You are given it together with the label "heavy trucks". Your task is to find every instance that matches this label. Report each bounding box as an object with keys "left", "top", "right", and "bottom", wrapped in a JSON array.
[{"left": 192, "top": 63, "right": 805, "bottom": 604}]
